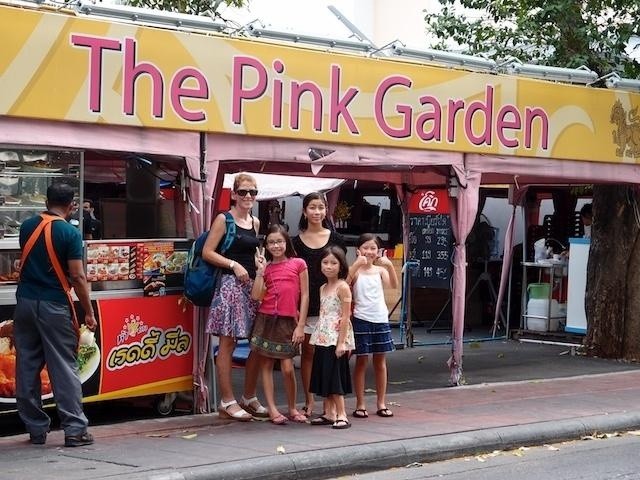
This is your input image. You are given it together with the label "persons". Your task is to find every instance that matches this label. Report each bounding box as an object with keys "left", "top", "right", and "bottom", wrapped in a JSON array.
[
  {"left": 252, "top": 225, "right": 309, "bottom": 424},
  {"left": 580, "top": 202, "right": 594, "bottom": 241},
  {"left": 202, "top": 174, "right": 272, "bottom": 423},
  {"left": 346, "top": 234, "right": 399, "bottom": 417},
  {"left": 312, "top": 246, "right": 355, "bottom": 429},
  {"left": 288, "top": 190, "right": 345, "bottom": 415},
  {"left": 18, "top": 184, "right": 104, "bottom": 448}
]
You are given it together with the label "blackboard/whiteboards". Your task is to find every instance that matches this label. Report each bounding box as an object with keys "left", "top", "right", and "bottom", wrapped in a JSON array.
[{"left": 408, "top": 214, "right": 451, "bottom": 289}]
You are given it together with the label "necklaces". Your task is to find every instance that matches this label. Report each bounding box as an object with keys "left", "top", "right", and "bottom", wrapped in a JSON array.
[{"left": 268, "top": 257, "right": 286, "bottom": 313}]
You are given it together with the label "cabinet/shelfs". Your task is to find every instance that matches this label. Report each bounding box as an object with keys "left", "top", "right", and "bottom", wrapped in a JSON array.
[
  {"left": 522, "top": 260, "right": 567, "bottom": 333},
  {"left": 0, "top": 146, "right": 84, "bottom": 285}
]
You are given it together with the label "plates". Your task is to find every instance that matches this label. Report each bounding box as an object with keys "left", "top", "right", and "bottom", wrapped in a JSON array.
[
  {"left": 87, "top": 247, "right": 129, "bottom": 278},
  {"left": 0, "top": 319, "right": 102, "bottom": 405},
  {"left": 0, "top": 158, "right": 61, "bottom": 237},
  {"left": 143, "top": 254, "right": 187, "bottom": 274}
]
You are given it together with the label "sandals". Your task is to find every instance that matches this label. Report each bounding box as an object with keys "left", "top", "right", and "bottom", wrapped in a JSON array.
[{"left": 218, "top": 394, "right": 393, "bottom": 429}]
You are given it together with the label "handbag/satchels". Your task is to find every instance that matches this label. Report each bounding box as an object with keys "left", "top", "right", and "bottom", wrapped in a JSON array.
[{"left": 183, "top": 230, "right": 218, "bottom": 307}]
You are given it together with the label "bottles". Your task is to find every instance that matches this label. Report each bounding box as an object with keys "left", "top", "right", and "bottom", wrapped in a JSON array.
[
  {"left": 394, "top": 241, "right": 404, "bottom": 258},
  {"left": 267, "top": 210, "right": 272, "bottom": 234},
  {"left": 553, "top": 252, "right": 558, "bottom": 261},
  {"left": 279, "top": 200, "right": 290, "bottom": 233}
]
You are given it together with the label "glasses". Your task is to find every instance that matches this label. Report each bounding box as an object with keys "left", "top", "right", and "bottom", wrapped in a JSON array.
[{"left": 234, "top": 188, "right": 259, "bottom": 197}]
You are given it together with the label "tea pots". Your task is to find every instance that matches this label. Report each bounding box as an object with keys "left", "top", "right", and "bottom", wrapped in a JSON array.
[{"left": 535, "top": 246, "right": 547, "bottom": 263}]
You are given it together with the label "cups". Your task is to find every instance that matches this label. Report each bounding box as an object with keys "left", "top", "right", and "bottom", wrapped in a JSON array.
[{"left": 388, "top": 249, "right": 394, "bottom": 258}]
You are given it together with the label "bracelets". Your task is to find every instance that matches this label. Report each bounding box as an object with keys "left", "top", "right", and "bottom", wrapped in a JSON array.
[{"left": 256, "top": 271, "right": 265, "bottom": 277}]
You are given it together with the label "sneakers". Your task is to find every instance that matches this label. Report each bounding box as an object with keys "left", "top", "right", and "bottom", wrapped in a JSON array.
[
  {"left": 64, "top": 432, "right": 95, "bottom": 446},
  {"left": 32, "top": 431, "right": 46, "bottom": 444}
]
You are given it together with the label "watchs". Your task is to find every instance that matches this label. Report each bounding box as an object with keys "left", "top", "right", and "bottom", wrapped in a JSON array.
[{"left": 230, "top": 261, "right": 235, "bottom": 270}]
